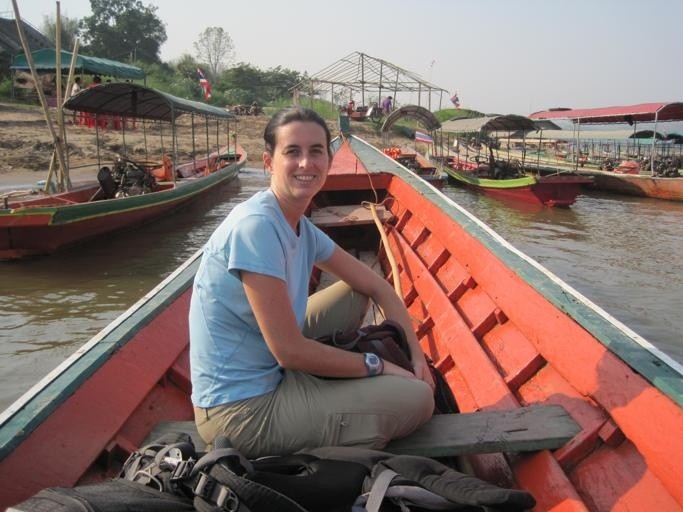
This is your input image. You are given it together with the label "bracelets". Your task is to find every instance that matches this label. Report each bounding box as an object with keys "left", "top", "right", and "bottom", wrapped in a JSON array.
[{"left": 377, "top": 357, "right": 384, "bottom": 375}]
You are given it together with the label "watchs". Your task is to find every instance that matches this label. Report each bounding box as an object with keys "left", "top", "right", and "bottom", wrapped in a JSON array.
[{"left": 363, "top": 352, "right": 380, "bottom": 377}]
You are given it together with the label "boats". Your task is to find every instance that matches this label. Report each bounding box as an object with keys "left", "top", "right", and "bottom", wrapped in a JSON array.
[
  {"left": 0, "top": 82, "right": 247, "bottom": 261},
  {"left": 0, "top": 134, "right": 683, "bottom": 511}
]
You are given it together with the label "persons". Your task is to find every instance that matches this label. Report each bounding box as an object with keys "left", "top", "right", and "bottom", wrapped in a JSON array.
[
  {"left": 70, "top": 75, "right": 130, "bottom": 125},
  {"left": 347, "top": 100, "right": 355, "bottom": 121},
  {"left": 188, "top": 106, "right": 437, "bottom": 460},
  {"left": 382, "top": 95, "right": 393, "bottom": 117}
]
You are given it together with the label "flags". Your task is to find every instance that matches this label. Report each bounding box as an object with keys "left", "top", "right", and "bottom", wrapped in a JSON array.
[
  {"left": 197, "top": 68, "right": 212, "bottom": 102},
  {"left": 414, "top": 130, "right": 433, "bottom": 145},
  {"left": 449, "top": 95, "right": 461, "bottom": 109}
]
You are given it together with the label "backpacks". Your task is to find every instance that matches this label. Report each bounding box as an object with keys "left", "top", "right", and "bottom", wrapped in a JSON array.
[{"left": 308, "top": 319, "right": 461, "bottom": 416}]
